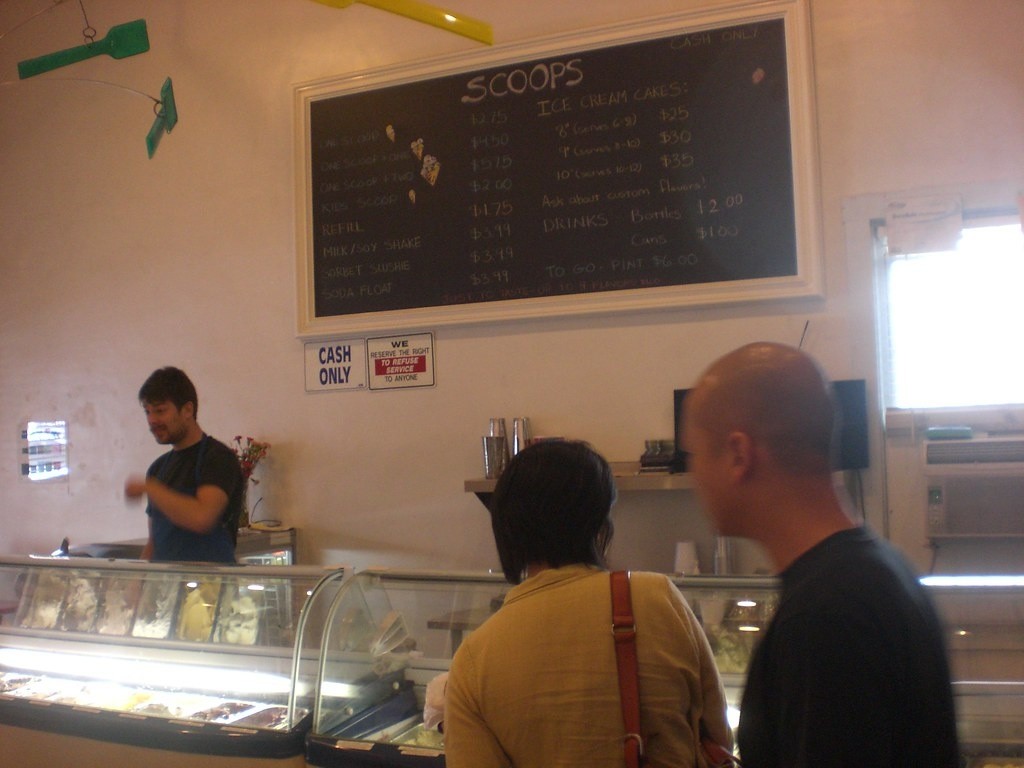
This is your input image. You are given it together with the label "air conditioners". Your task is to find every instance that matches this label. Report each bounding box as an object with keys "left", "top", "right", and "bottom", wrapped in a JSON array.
[{"left": 921, "top": 434, "right": 1024, "bottom": 539}]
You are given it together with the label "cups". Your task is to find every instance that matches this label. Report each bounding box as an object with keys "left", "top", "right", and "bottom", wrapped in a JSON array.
[
  {"left": 674, "top": 541, "right": 702, "bottom": 576},
  {"left": 481, "top": 416, "right": 532, "bottom": 480},
  {"left": 713, "top": 536, "right": 738, "bottom": 577}
]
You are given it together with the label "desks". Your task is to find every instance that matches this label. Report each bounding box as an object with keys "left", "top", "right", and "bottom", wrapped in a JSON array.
[{"left": 427, "top": 609, "right": 494, "bottom": 659}]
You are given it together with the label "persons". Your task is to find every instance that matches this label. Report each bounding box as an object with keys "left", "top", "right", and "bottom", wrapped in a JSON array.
[
  {"left": 685, "top": 341, "right": 967, "bottom": 768},
  {"left": 124, "top": 367, "right": 244, "bottom": 563},
  {"left": 444, "top": 439, "right": 734, "bottom": 768}
]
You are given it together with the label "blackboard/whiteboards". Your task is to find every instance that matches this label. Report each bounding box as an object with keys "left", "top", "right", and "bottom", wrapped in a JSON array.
[{"left": 293, "top": 0, "right": 828, "bottom": 340}]
